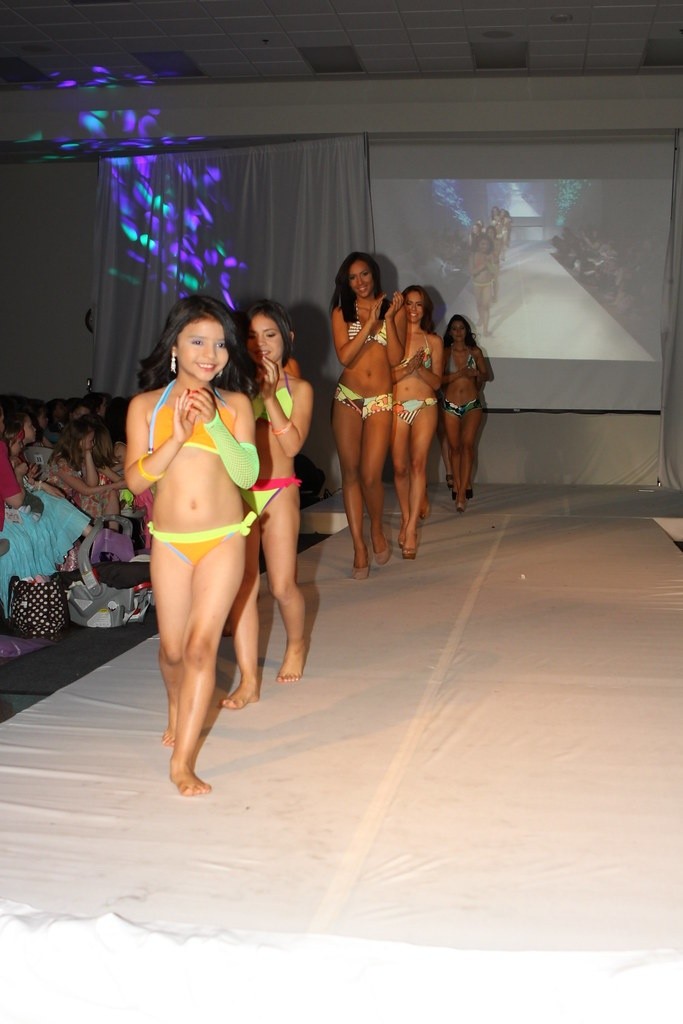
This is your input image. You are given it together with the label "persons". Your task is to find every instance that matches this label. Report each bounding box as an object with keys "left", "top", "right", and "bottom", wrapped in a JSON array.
[
  {"left": 218, "top": 300, "right": 311, "bottom": 709},
  {"left": 0, "top": 385, "right": 151, "bottom": 622},
  {"left": 548, "top": 210, "right": 661, "bottom": 330},
  {"left": 384, "top": 284, "right": 443, "bottom": 561},
  {"left": 438, "top": 422, "right": 454, "bottom": 490},
  {"left": 458, "top": 196, "right": 515, "bottom": 329},
  {"left": 330, "top": 251, "right": 406, "bottom": 580},
  {"left": 436, "top": 315, "right": 487, "bottom": 510},
  {"left": 446, "top": 447, "right": 473, "bottom": 499},
  {"left": 416, "top": 473, "right": 432, "bottom": 519},
  {"left": 124, "top": 293, "right": 262, "bottom": 797}
]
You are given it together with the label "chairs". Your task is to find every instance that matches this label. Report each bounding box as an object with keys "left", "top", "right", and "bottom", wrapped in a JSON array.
[{"left": 23, "top": 446, "right": 57, "bottom": 481}]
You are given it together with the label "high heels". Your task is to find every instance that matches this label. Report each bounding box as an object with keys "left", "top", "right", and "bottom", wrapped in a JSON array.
[
  {"left": 402, "top": 528, "right": 417, "bottom": 559},
  {"left": 465, "top": 482, "right": 473, "bottom": 499},
  {"left": 455, "top": 499, "right": 466, "bottom": 514},
  {"left": 451, "top": 488, "right": 459, "bottom": 499},
  {"left": 398, "top": 525, "right": 407, "bottom": 548},
  {"left": 446, "top": 476, "right": 455, "bottom": 489},
  {"left": 419, "top": 497, "right": 430, "bottom": 519},
  {"left": 370, "top": 532, "right": 390, "bottom": 566},
  {"left": 352, "top": 545, "right": 370, "bottom": 580}
]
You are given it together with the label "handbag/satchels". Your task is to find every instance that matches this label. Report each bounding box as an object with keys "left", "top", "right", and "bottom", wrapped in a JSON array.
[{"left": 7, "top": 573, "right": 71, "bottom": 641}]
